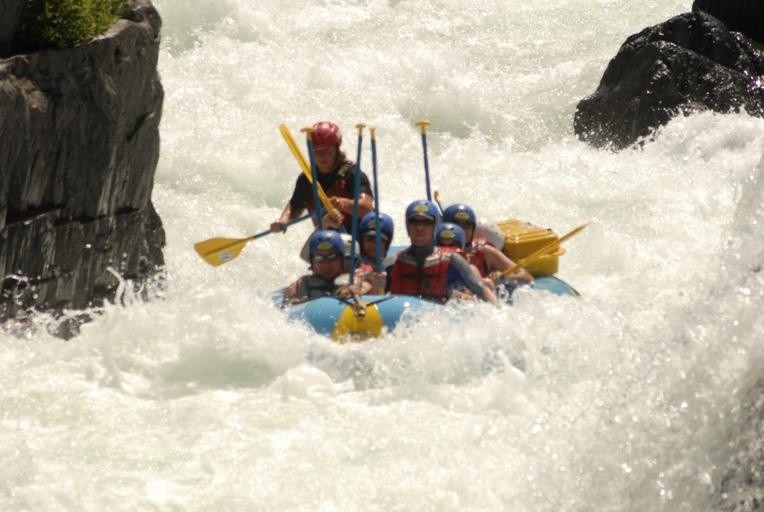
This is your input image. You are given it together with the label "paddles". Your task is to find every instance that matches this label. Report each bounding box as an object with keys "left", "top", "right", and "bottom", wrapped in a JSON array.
[
  {"left": 494, "top": 222, "right": 590, "bottom": 285},
  {"left": 280, "top": 126, "right": 349, "bottom": 233},
  {"left": 193, "top": 206, "right": 327, "bottom": 266}
]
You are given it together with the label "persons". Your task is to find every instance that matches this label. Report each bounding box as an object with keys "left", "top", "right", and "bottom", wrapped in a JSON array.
[
  {"left": 271, "top": 120, "right": 374, "bottom": 232},
  {"left": 384, "top": 200, "right": 502, "bottom": 304},
  {"left": 339, "top": 232, "right": 387, "bottom": 294},
  {"left": 300, "top": 209, "right": 394, "bottom": 294},
  {"left": 443, "top": 203, "right": 535, "bottom": 287},
  {"left": 436, "top": 222, "right": 494, "bottom": 301},
  {"left": 283, "top": 230, "right": 373, "bottom": 306}
]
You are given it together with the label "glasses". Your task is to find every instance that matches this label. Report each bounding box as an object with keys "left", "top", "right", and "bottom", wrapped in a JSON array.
[{"left": 313, "top": 257, "right": 342, "bottom": 263}]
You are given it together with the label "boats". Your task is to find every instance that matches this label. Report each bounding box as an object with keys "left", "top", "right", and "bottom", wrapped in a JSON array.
[{"left": 270, "top": 248, "right": 582, "bottom": 335}]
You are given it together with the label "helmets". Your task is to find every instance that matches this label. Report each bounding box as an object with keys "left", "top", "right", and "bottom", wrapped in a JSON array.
[
  {"left": 309, "top": 200, "right": 477, "bottom": 256},
  {"left": 307, "top": 122, "right": 342, "bottom": 149}
]
